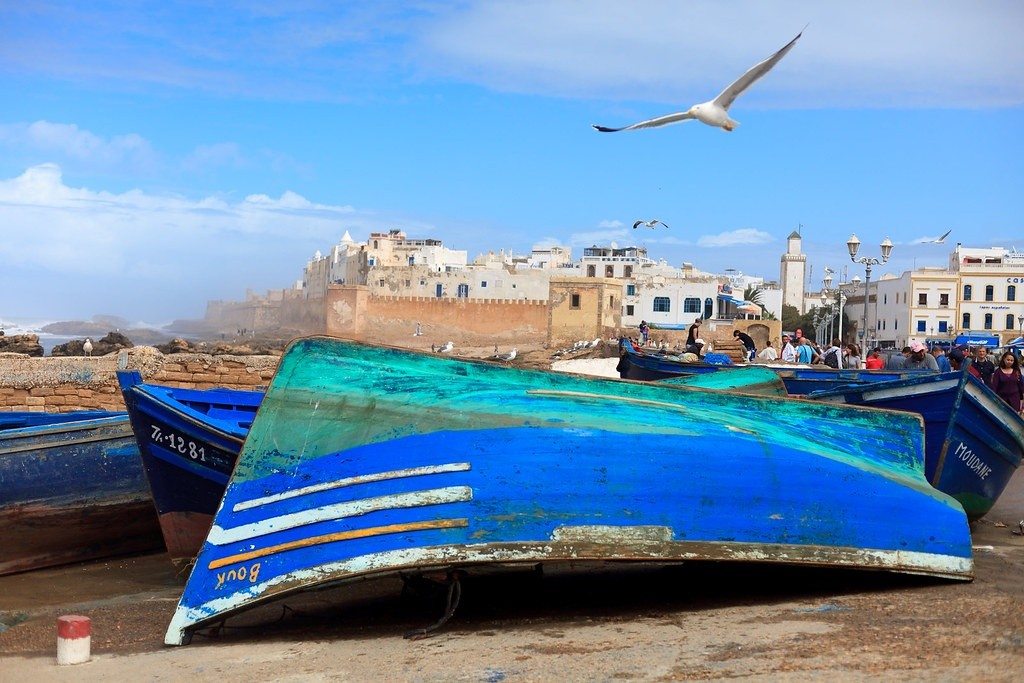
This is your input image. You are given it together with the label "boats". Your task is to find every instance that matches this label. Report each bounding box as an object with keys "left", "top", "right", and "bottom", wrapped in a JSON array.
[
  {"left": 617, "top": 336, "right": 1024, "bottom": 524},
  {"left": 164, "top": 338, "right": 978, "bottom": 650},
  {"left": 114, "top": 367, "right": 266, "bottom": 575},
  {"left": 0, "top": 409, "right": 168, "bottom": 576}
]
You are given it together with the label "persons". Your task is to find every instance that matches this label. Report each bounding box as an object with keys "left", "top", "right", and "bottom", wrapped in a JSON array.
[
  {"left": 797, "top": 337, "right": 825, "bottom": 364},
  {"left": 757, "top": 340, "right": 778, "bottom": 361},
  {"left": 733, "top": 330, "right": 756, "bottom": 361},
  {"left": 992, "top": 351, "right": 1024, "bottom": 415},
  {"left": 971, "top": 345, "right": 995, "bottom": 388},
  {"left": 624, "top": 320, "right": 682, "bottom": 349},
  {"left": 949, "top": 350, "right": 984, "bottom": 383},
  {"left": 687, "top": 318, "right": 703, "bottom": 345},
  {"left": 781, "top": 328, "right": 1024, "bottom": 400},
  {"left": 887, "top": 347, "right": 911, "bottom": 368},
  {"left": 658, "top": 345, "right": 667, "bottom": 355},
  {"left": 866, "top": 347, "right": 885, "bottom": 369},
  {"left": 903, "top": 342, "right": 940, "bottom": 370},
  {"left": 686, "top": 338, "right": 706, "bottom": 359},
  {"left": 931, "top": 345, "right": 951, "bottom": 372}
]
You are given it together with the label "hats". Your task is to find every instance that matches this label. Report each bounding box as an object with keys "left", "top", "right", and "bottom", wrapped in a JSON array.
[
  {"left": 695, "top": 339, "right": 706, "bottom": 344},
  {"left": 945, "top": 348, "right": 964, "bottom": 360},
  {"left": 695, "top": 318, "right": 702, "bottom": 324},
  {"left": 911, "top": 342, "right": 925, "bottom": 353}
]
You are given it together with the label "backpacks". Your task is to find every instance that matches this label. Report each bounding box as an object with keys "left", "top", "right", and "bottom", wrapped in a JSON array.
[{"left": 824, "top": 349, "right": 839, "bottom": 369}]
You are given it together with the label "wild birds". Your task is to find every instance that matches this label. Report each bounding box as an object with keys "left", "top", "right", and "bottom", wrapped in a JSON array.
[
  {"left": 921, "top": 229, "right": 952, "bottom": 245},
  {"left": 437, "top": 341, "right": 455, "bottom": 353},
  {"left": 633, "top": 220, "right": 669, "bottom": 229},
  {"left": 824, "top": 266, "right": 837, "bottom": 275},
  {"left": 83, "top": 338, "right": 94, "bottom": 356},
  {"left": 493, "top": 348, "right": 519, "bottom": 365},
  {"left": 592, "top": 22, "right": 809, "bottom": 133}
]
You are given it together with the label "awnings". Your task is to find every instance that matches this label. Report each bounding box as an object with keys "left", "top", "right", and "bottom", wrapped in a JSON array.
[
  {"left": 956, "top": 334, "right": 999, "bottom": 347},
  {"left": 719, "top": 294, "right": 750, "bottom": 306},
  {"left": 926, "top": 338, "right": 955, "bottom": 344}
]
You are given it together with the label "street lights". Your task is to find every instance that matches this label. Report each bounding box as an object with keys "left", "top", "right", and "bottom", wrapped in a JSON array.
[
  {"left": 948, "top": 324, "right": 954, "bottom": 354},
  {"left": 811, "top": 291, "right": 848, "bottom": 352},
  {"left": 929, "top": 327, "right": 934, "bottom": 355},
  {"left": 1018, "top": 315, "right": 1024, "bottom": 338},
  {"left": 845, "top": 233, "right": 895, "bottom": 369},
  {"left": 967, "top": 328, "right": 972, "bottom": 354},
  {"left": 823, "top": 272, "right": 861, "bottom": 343}
]
